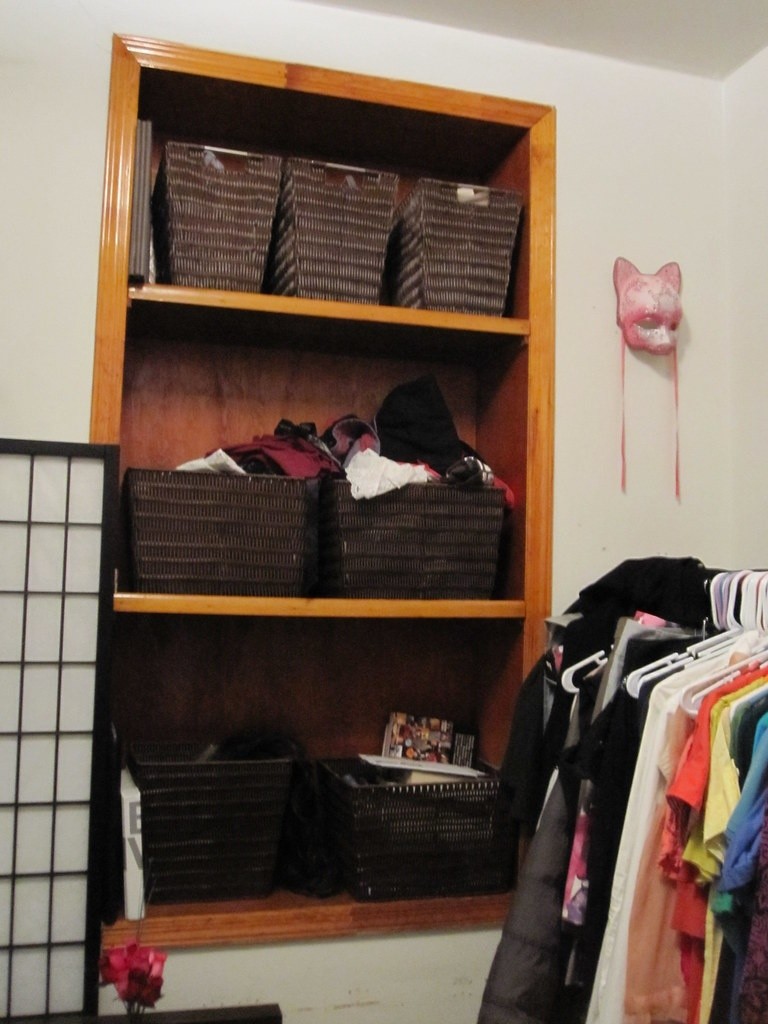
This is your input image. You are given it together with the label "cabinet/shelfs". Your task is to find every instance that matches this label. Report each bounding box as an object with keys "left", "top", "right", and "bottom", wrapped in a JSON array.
[{"left": 89, "top": 35, "right": 557, "bottom": 955}]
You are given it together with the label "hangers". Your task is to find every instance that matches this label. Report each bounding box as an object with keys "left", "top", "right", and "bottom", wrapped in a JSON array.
[{"left": 561, "top": 570, "right": 768, "bottom": 715}]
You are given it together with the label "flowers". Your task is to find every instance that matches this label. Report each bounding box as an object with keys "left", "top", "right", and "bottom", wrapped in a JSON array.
[{"left": 99, "top": 940, "right": 167, "bottom": 1024}]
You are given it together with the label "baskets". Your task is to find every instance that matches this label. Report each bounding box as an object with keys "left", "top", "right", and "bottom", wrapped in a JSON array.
[
  {"left": 319, "top": 478, "right": 504, "bottom": 601},
  {"left": 130, "top": 741, "right": 295, "bottom": 906},
  {"left": 121, "top": 467, "right": 322, "bottom": 601},
  {"left": 270, "top": 157, "right": 401, "bottom": 306},
  {"left": 316, "top": 757, "right": 503, "bottom": 902},
  {"left": 385, "top": 178, "right": 523, "bottom": 318},
  {"left": 153, "top": 142, "right": 283, "bottom": 293}
]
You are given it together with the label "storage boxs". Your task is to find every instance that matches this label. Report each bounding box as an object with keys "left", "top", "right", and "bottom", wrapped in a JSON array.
[
  {"left": 316, "top": 757, "right": 508, "bottom": 905},
  {"left": 126, "top": 738, "right": 295, "bottom": 905},
  {"left": 267, "top": 159, "right": 399, "bottom": 305},
  {"left": 388, "top": 176, "right": 522, "bottom": 317},
  {"left": 151, "top": 142, "right": 282, "bottom": 296},
  {"left": 319, "top": 478, "right": 504, "bottom": 601},
  {"left": 120, "top": 467, "right": 317, "bottom": 596}
]
